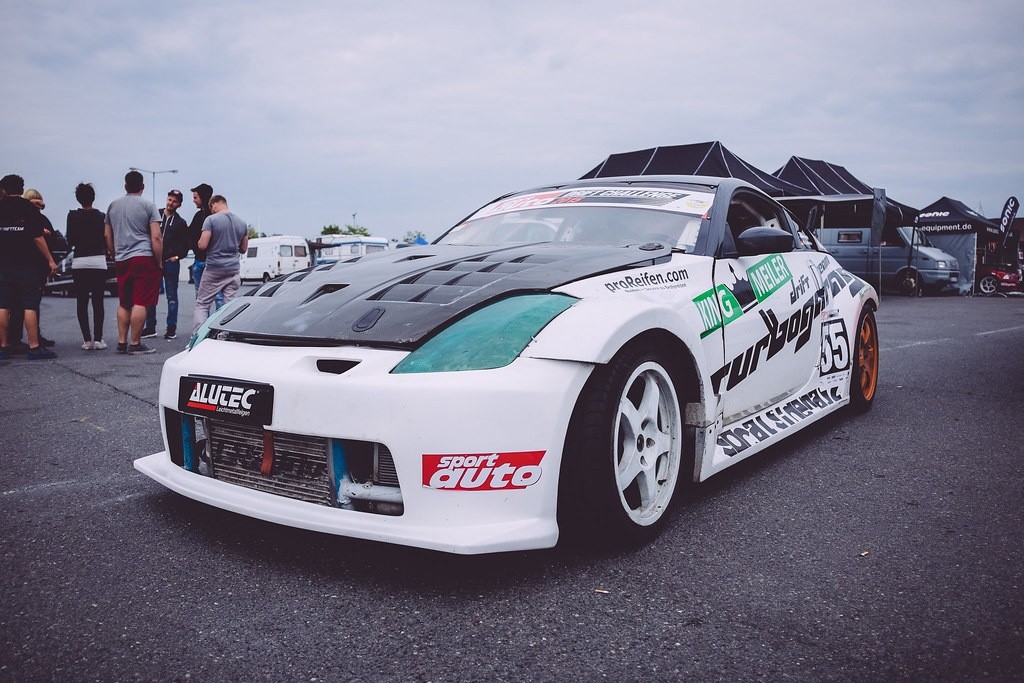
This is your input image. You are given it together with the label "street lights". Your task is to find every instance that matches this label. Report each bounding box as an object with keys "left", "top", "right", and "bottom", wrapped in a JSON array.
[{"left": 130, "top": 166, "right": 178, "bottom": 208}]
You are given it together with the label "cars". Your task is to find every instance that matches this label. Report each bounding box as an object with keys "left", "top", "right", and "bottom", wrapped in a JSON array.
[
  {"left": 974, "top": 251, "right": 1019, "bottom": 295},
  {"left": 134, "top": 175, "right": 881, "bottom": 551},
  {"left": 41, "top": 248, "right": 120, "bottom": 298}
]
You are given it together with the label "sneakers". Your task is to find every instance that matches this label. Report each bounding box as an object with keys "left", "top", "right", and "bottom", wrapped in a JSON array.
[
  {"left": 116, "top": 343, "right": 128, "bottom": 353},
  {"left": 164, "top": 329, "right": 176, "bottom": 339},
  {"left": 128, "top": 342, "right": 156, "bottom": 355},
  {"left": 140, "top": 328, "right": 157, "bottom": 338}
]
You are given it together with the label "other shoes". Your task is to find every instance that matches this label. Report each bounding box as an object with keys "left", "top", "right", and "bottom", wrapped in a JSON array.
[
  {"left": 82, "top": 339, "right": 92, "bottom": 349},
  {"left": 38, "top": 334, "right": 55, "bottom": 347},
  {"left": 0, "top": 347, "right": 9, "bottom": 361},
  {"left": 7, "top": 342, "right": 30, "bottom": 354},
  {"left": 94, "top": 340, "right": 107, "bottom": 349},
  {"left": 26, "top": 347, "right": 57, "bottom": 359}
]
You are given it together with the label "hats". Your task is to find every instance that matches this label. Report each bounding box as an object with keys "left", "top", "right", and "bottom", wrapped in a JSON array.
[{"left": 168, "top": 190, "right": 183, "bottom": 203}]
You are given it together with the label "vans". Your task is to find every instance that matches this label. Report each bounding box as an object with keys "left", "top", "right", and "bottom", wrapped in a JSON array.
[
  {"left": 314, "top": 235, "right": 388, "bottom": 267},
  {"left": 811, "top": 225, "right": 960, "bottom": 295},
  {"left": 239, "top": 235, "right": 311, "bottom": 284}
]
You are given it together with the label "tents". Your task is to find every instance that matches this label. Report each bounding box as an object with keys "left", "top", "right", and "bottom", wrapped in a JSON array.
[
  {"left": 913, "top": 197, "right": 1021, "bottom": 297},
  {"left": 578, "top": 140, "right": 921, "bottom": 302}
]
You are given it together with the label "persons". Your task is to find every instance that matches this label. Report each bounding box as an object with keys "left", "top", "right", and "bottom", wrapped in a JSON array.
[
  {"left": 7, "top": 189, "right": 56, "bottom": 352},
  {"left": 103, "top": 171, "right": 163, "bottom": 354},
  {"left": 141, "top": 190, "right": 189, "bottom": 339},
  {"left": 66, "top": 183, "right": 115, "bottom": 350},
  {"left": 185, "top": 195, "right": 248, "bottom": 350},
  {"left": 188, "top": 183, "right": 225, "bottom": 318},
  {"left": 0, "top": 174, "right": 59, "bottom": 360}
]
